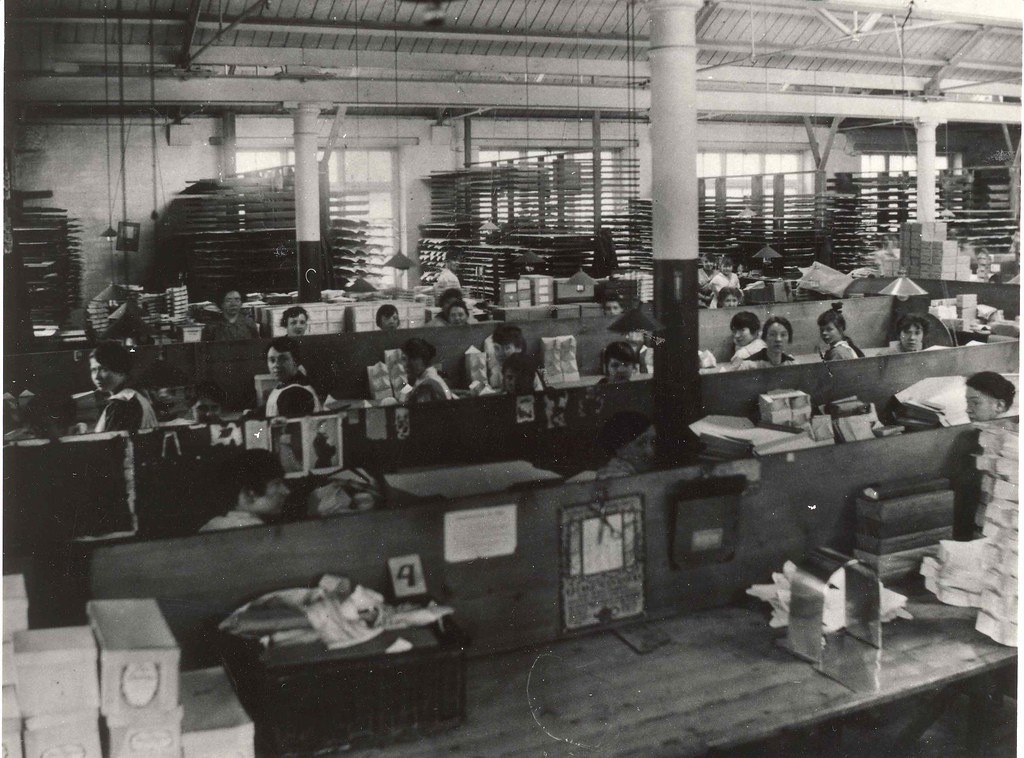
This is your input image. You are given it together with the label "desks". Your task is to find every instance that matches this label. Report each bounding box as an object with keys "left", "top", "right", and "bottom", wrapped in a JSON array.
[{"left": 292, "top": 563, "right": 1017, "bottom": 758}]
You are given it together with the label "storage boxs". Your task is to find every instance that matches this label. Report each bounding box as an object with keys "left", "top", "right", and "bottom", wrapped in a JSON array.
[
  {"left": 84, "top": 281, "right": 425, "bottom": 354},
  {"left": 878, "top": 220, "right": 1020, "bottom": 285},
  {"left": 856, "top": 425, "right": 1019, "bottom": 647},
  {"left": 760, "top": 389, "right": 810, "bottom": 427},
  {"left": 487, "top": 274, "right": 653, "bottom": 320},
  {"left": 3, "top": 572, "right": 470, "bottom": 758}
]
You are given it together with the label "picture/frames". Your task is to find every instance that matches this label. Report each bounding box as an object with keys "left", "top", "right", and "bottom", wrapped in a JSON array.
[{"left": 271, "top": 414, "right": 344, "bottom": 480}]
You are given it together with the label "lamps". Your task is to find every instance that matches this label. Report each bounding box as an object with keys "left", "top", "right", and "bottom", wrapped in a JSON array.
[
  {"left": 740, "top": 60, "right": 758, "bottom": 219},
  {"left": 513, "top": 0, "right": 548, "bottom": 271},
  {"left": 101, "top": 9, "right": 117, "bottom": 242},
  {"left": 877, "top": 0, "right": 930, "bottom": 301},
  {"left": 383, "top": 0, "right": 415, "bottom": 277},
  {"left": 753, "top": 1, "right": 784, "bottom": 265}
]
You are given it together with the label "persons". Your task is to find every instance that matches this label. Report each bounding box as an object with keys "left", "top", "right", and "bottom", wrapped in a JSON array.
[
  {"left": 818, "top": 301, "right": 864, "bottom": 361},
  {"left": 280, "top": 308, "right": 309, "bottom": 337},
  {"left": 965, "top": 370, "right": 1015, "bottom": 423},
  {"left": 730, "top": 311, "right": 803, "bottom": 372},
  {"left": 196, "top": 458, "right": 294, "bottom": 535},
  {"left": 200, "top": 286, "right": 258, "bottom": 346},
  {"left": 872, "top": 315, "right": 931, "bottom": 358},
  {"left": 424, "top": 247, "right": 469, "bottom": 327},
  {"left": 191, "top": 381, "right": 227, "bottom": 426},
  {"left": 376, "top": 305, "right": 400, "bottom": 330},
  {"left": 397, "top": 336, "right": 465, "bottom": 403},
  {"left": 479, "top": 323, "right": 549, "bottom": 395},
  {"left": 594, "top": 341, "right": 638, "bottom": 385},
  {"left": 233, "top": 336, "right": 325, "bottom": 424},
  {"left": 603, "top": 294, "right": 624, "bottom": 317},
  {"left": 87, "top": 337, "right": 161, "bottom": 434},
  {"left": 697, "top": 252, "right": 744, "bottom": 309}
]
